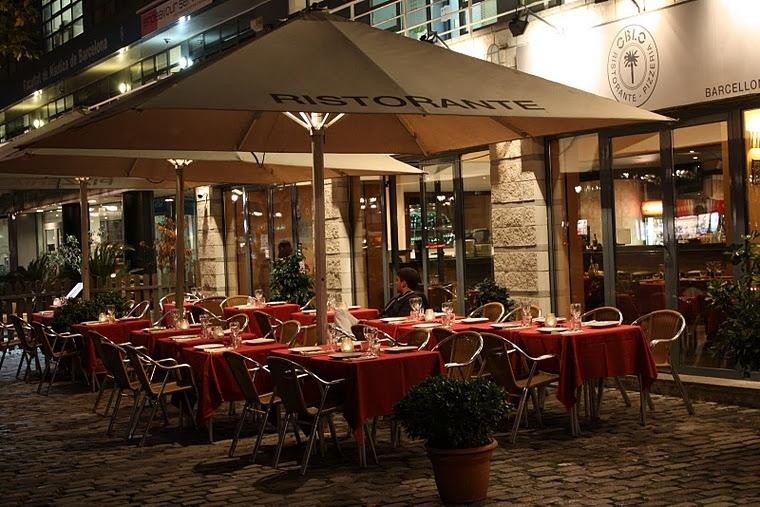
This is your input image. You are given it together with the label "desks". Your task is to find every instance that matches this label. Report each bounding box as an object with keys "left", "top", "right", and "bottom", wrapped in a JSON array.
[
  {"left": 389, "top": 255, "right": 494, "bottom": 289},
  {"left": 585, "top": 241, "right": 726, "bottom": 274}
]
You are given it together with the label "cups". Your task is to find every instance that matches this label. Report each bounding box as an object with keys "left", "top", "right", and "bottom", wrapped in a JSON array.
[
  {"left": 363, "top": 326, "right": 378, "bottom": 354},
  {"left": 230, "top": 322, "right": 240, "bottom": 350},
  {"left": 522, "top": 299, "right": 532, "bottom": 327},
  {"left": 570, "top": 304, "right": 581, "bottom": 331},
  {"left": 409, "top": 298, "right": 422, "bottom": 320},
  {"left": 199, "top": 315, "right": 209, "bottom": 338},
  {"left": 255, "top": 289, "right": 263, "bottom": 309},
  {"left": 171, "top": 309, "right": 179, "bottom": 330},
  {"left": 327, "top": 323, "right": 336, "bottom": 351},
  {"left": 192, "top": 288, "right": 197, "bottom": 303},
  {"left": 442, "top": 302, "right": 453, "bottom": 327},
  {"left": 197, "top": 288, "right": 202, "bottom": 302},
  {"left": 107, "top": 304, "right": 115, "bottom": 324}
]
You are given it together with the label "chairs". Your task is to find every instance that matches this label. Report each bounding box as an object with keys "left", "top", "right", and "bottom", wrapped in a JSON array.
[
  {"left": 327, "top": 322, "right": 359, "bottom": 342},
  {"left": 251, "top": 356, "right": 380, "bottom": 476},
  {"left": 128, "top": 302, "right": 151, "bottom": 322},
  {"left": 630, "top": 309, "right": 695, "bottom": 417},
  {"left": 221, "top": 295, "right": 256, "bottom": 316},
  {"left": 153, "top": 310, "right": 180, "bottom": 328},
  {"left": 31, "top": 320, "right": 89, "bottom": 396},
  {"left": 0, "top": 320, "right": 32, "bottom": 374},
  {"left": 161, "top": 291, "right": 200, "bottom": 312},
  {"left": 266, "top": 321, "right": 300, "bottom": 347},
  {"left": 349, "top": 324, "right": 409, "bottom": 346},
  {"left": 473, "top": 331, "right": 560, "bottom": 444},
  {"left": 363, "top": 328, "right": 432, "bottom": 442},
  {"left": 580, "top": 305, "right": 631, "bottom": 418},
  {"left": 14, "top": 313, "right": 51, "bottom": 382},
  {"left": 106, "top": 341, "right": 170, "bottom": 437},
  {"left": 501, "top": 304, "right": 558, "bottom": 410},
  {"left": 434, "top": 327, "right": 492, "bottom": 378},
  {"left": 222, "top": 349, "right": 301, "bottom": 464},
  {"left": 224, "top": 314, "right": 250, "bottom": 333},
  {"left": 300, "top": 295, "right": 336, "bottom": 313},
  {"left": 118, "top": 300, "right": 134, "bottom": 317},
  {"left": 467, "top": 303, "right": 505, "bottom": 325},
  {"left": 92, "top": 329, "right": 153, "bottom": 415},
  {"left": 254, "top": 309, "right": 284, "bottom": 339},
  {"left": 196, "top": 295, "right": 227, "bottom": 319},
  {"left": 192, "top": 304, "right": 221, "bottom": 326},
  {"left": 295, "top": 322, "right": 317, "bottom": 346},
  {"left": 126, "top": 345, "right": 200, "bottom": 447},
  {"left": 390, "top": 332, "right": 481, "bottom": 449}
]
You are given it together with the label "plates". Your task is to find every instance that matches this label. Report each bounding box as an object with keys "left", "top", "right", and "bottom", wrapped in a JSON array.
[
  {"left": 490, "top": 322, "right": 522, "bottom": 328},
  {"left": 266, "top": 302, "right": 286, "bottom": 304},
  {"left": 585, "top": 321, "right": 619, "bottom": 325},
  {"left": 380, "top": 317, "right": 407, "bottom": 322},
  {"left": 385, "top": 347, "right": 418, "bottom": 350},
  {"left": 245, "top": 339, "right": 274, "bottom": 343},
  {"left": 462, "top": 319, "right": 488, "bottom": 322},
  {"left": 538, "top": 328, "right": 569, "bottom": 331},
  {"left": 328, "top": 353, "right": 366, "bottom": 357},
  {"left": 414, "top": 324, "right": 444, "bottom": 327},
  {"left": 288, "top": 347, "right": 322, "bottom": 351},
  {"left": 536, "top": 316, "right": 568, "bottom": 321},
  {"left": 194, "top": 344, "right": 224, "bottom": 348}
]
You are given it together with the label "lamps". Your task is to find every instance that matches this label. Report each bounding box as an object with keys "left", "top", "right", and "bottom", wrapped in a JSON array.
[
  {"left": 369, "top": 192, "right": 376, "bottom": 209},
  {"left": 420, "top": 31, "right": 437, "bottom": 51},
  {"left": 252, "top": 209, "right": 263, "bottom": 216},
  {"left": 360, "top": 192, "right": 365, "bottom": 210},
  {"left": 230, "top": 188, "right": 243, "bottom": 202},
  {"left": 273, "top": 212, "right": 283, "bottom": 218},
  {"left": 508, "top": 8, "right": 530, "bottom": 37},
  {"left": 744, "top": 108, "right": 760, "bottom": 184}
]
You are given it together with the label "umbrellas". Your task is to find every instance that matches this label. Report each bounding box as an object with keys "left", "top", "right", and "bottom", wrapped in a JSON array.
[
  {"left": 3, "top": 107, "right": 96, "bottom": 304},
  {"left": 0, "top": 148, "right": 429, "bottom": 325},
  {"left": 14, "top": 6, "right": 679, "bottom": 345}
]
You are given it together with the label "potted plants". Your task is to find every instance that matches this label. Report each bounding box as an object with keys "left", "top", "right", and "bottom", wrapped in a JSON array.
[
  {"left": 393, "top": 376, "right": 512, "bottom": 505},
  {"left": 705, "top": 230, "right": 760, "bottom": 373}
]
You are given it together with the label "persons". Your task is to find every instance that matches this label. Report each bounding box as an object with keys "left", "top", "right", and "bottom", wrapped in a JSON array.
[{"left": 334, "top": 266, "right": 427, "bottom": 338}]
